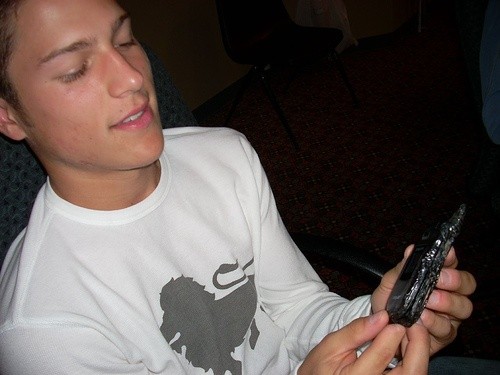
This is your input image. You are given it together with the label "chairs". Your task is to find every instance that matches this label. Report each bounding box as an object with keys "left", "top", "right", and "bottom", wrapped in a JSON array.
[
  {"left": 215, "top": 1, "right": 362, "bottom": 153},
  {"left": 1, "top": 39, "right": 386, "bottom": 291}
]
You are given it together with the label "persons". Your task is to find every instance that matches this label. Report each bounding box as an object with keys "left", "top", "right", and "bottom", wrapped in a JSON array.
[{"left": 1, "top": 0, "right": 477, "bottom": 375}]
